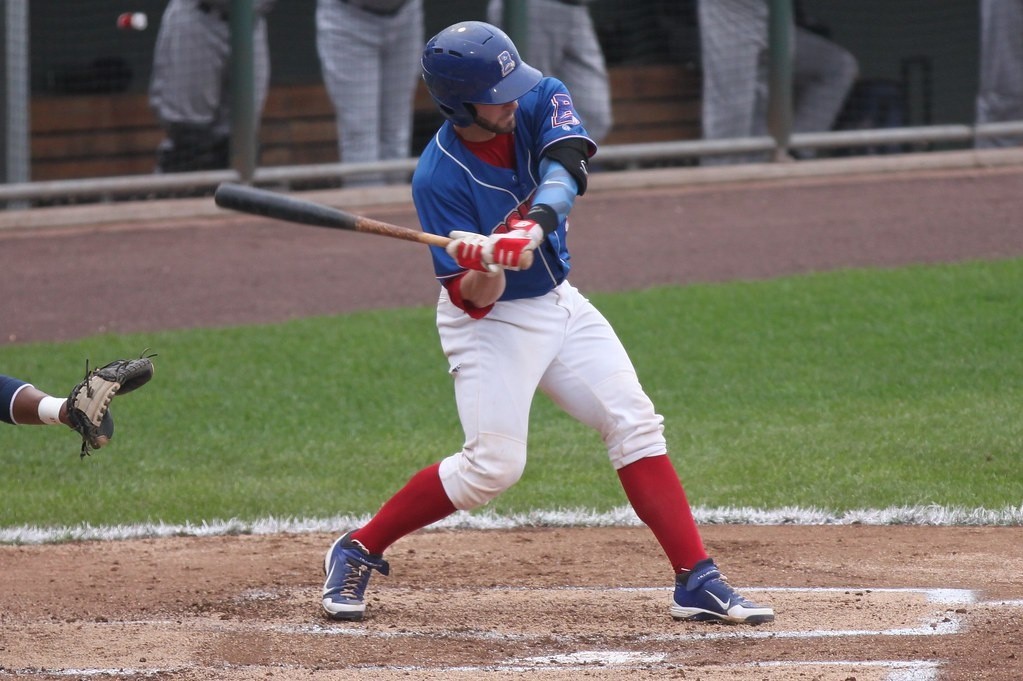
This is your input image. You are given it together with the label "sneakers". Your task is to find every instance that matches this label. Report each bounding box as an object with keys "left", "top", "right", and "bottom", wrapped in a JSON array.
[
  {"left": 670, "top": 560, "right": 774, "bottom": 626},
  {"left": 323, "top": 527, "right": 391, "bottom": 621}
]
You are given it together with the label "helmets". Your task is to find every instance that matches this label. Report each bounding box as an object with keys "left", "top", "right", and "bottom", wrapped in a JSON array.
[{"left": 422, "top": 21, "right": 543, "bottom": 128}]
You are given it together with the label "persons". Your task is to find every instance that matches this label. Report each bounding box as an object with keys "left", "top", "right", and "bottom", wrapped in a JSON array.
[
  {"left": 151, "top": 1, "right": 270, "bottom": 169},
  {"left": 699, "top": 0, "right": 859, "bottom": 163},
  {"left": 0, "top": 348, "right": 157, "bottom": 460},
  {"left": 526, "top": 0, "right": 614, "bottom": 143},
  {"left": 322, "top": 17, "right": 776, "bottom": 626},
  {"left": 316, "top": 0, "right": 425, "bottom": 185}
]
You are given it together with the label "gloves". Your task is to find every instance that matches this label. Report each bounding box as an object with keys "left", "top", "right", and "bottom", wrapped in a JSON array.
[
  {"left": 446, "top": 230, "right": 510, "bottom": 277},
  {"left": 483, "top": 218, "right": 544, "bottom": 271}
]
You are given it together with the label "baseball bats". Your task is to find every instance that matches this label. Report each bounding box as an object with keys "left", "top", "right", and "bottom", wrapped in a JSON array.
[{"left": 213, "top": 181, "right": 535, "bottom": 271}]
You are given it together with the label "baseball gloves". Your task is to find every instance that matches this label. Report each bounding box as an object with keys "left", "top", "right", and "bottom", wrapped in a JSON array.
[{"left": 64, "top": 357, "right": 156, "bottom": 450}]
